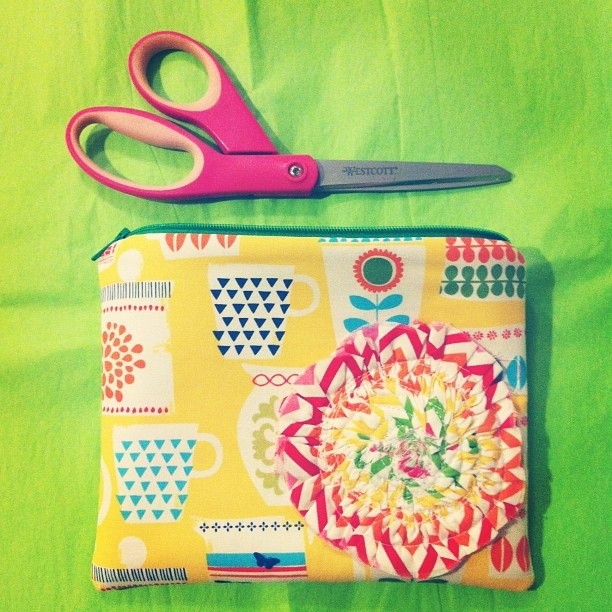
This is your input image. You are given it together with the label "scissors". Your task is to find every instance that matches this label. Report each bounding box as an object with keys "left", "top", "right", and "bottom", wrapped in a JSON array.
[{"left": 66, "top": 31, "right": 513, "bottom": 202}]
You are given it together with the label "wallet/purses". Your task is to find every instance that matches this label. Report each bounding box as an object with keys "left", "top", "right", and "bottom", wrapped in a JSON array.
[{"left": 89, "top": 221, "right": 536, "bottom": 593}]
